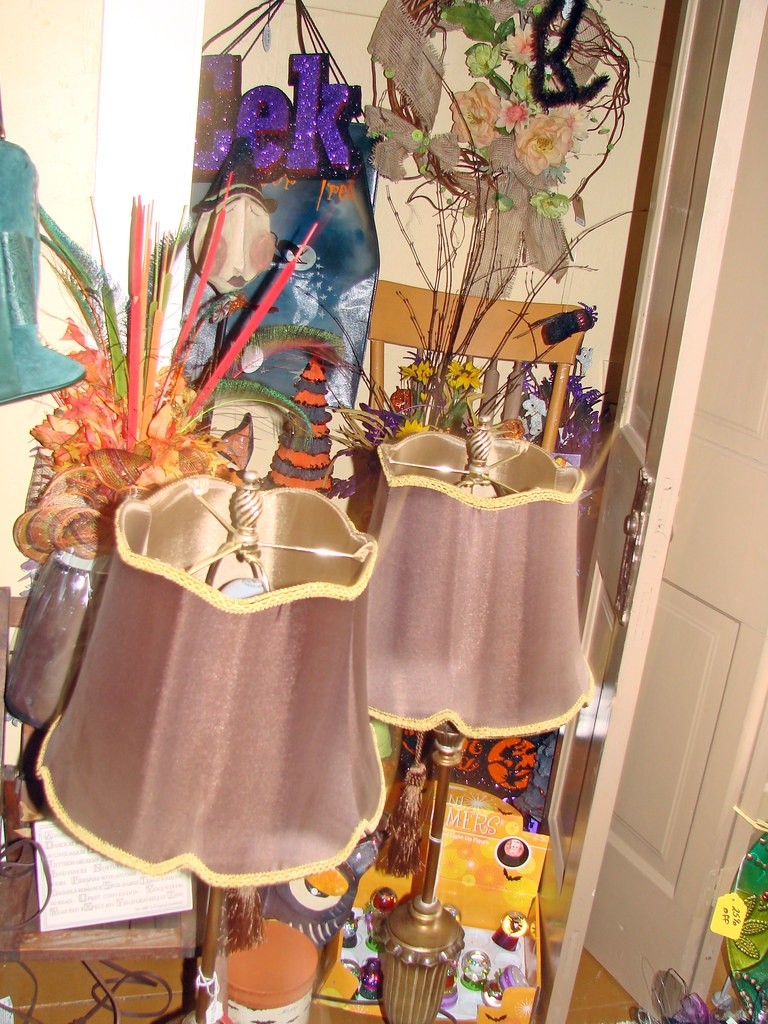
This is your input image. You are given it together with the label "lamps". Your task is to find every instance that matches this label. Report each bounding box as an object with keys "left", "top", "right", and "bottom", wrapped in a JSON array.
[
  {"left": 366, "top": 423, "right": 597, "bottom": 1024},
  {"left": 34, "top": 469, "right": 387, "bottom": 1024},
  {"left": 0, "top": 140, "right": 87, "bottom": 405}
]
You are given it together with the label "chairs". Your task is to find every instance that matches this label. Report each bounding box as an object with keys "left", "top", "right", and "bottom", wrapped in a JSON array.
[
  {"left": 1, "top": 585, "right": 198, "bottom": 961},
  {"left": 365, "top": 280, "right": 586, "bottom": 454}
]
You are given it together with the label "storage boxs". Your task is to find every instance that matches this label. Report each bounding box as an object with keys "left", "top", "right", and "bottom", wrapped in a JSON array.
[{"left": 309, "top": 780, "right": 551, "bottom": 1024}]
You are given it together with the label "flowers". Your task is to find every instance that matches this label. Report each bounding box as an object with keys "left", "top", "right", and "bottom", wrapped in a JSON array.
[
  {"left": 369, "top": 0, "right": 618, "bottom": 219},
  {"left": 329, "top": 356, "right": 516, "bottom": 501},
  {"left": 12, "top": 170, "right": 348, "bottom": 563}
]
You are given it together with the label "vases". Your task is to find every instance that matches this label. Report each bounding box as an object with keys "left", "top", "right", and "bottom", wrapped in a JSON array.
[{"left": 3, "top": 545, "right": 115, "bottom": 729}]
